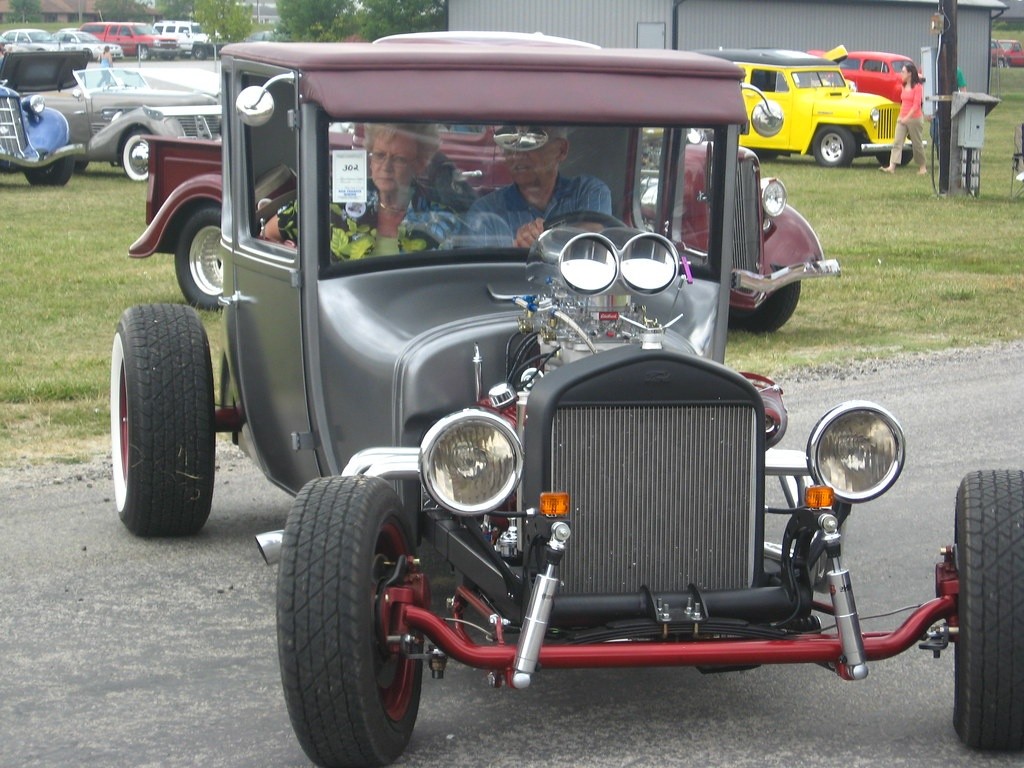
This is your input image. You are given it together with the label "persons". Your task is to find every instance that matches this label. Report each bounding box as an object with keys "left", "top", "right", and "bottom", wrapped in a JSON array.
[
  {"left": 879, "top": 61, "right": 927, "bottom": 173},
  {"left": 258, "top": 118, "right": 612, "bottom": 259},
  {"left": 96, "top": 45, "right": 113, "bottom": 87},
  {"left": 930, "top": 65, "right": 967, "bottom": 159}
]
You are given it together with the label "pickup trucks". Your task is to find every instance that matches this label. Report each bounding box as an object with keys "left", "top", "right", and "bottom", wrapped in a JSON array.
[{"left": 131, "top": 33, "right": 842, "bottom": 311}]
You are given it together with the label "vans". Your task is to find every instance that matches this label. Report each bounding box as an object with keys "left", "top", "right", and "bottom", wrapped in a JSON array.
[{"left": 154, "top": 20, "right": 231, "bottom": 60}]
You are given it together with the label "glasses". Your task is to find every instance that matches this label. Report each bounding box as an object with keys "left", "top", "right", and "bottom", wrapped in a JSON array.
[{"left": 369, "top": 152, "right": 421, "bottom": 167}]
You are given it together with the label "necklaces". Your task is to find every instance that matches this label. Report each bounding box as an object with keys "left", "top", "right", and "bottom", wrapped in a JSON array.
[{"left": 381, "top": 202, "right": 408, "bottom": 215}]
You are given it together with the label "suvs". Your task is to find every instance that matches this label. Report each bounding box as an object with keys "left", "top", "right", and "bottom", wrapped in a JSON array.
[
  {"left": 79, "top": 22, "right": 179, "bottom": 60},
  {"left": 110, "top": 30, "right": 1023, "bottom": 768}
]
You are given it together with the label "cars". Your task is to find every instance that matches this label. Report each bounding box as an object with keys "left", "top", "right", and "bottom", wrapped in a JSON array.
[
  {"left": 992, "top": 39, "right": 1024, "bottom": 68},
  {"left": 0, "top": 79, "right": 84, "bottom": 186},
  {"left": 642, "top": 44, "right": 927, "bottom": 167},
  {"left": 805, "top": 49, "right": 920, "bottom": 102},
  {"left": 52, "top": 28, "right": 125, "bottom": 62},
  {"left": 0, "top": 51, "right": 221, "bottom": 182},
  {"left": 0, "top": 29, "right": 64, "bottom": 51}
]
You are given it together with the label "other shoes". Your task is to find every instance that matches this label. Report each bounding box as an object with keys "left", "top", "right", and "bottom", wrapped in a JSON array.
[{"left": 879, "top": 167, "right": 893, "bottom": 174}]
